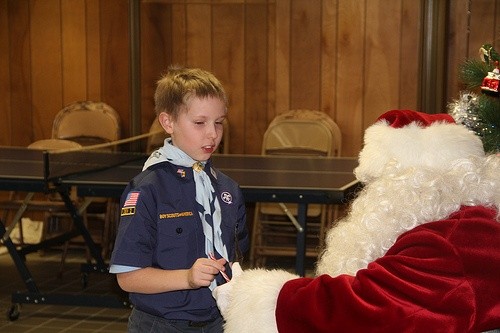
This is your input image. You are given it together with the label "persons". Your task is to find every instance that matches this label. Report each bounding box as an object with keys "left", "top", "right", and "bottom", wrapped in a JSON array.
[
  {"left": 109, "top": 68, "right": 251, "bottom": 333},
  {"left": 212, "top": 107, "right": 500, "bottom": 333}
]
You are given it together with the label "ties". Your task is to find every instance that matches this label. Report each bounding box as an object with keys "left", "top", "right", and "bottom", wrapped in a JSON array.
[{"left": 142, "top": 138, "right": 229, "bottom": 263}]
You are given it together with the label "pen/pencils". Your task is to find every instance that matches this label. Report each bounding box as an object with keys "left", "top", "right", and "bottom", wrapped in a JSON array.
[{"left": 208, "top": 252, "right": 230, "bottom": 282}]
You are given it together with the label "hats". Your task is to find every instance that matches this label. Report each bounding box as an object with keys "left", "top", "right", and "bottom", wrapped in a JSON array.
[{"left": 353, "top": 110, "right": 485, "bottom": 183}]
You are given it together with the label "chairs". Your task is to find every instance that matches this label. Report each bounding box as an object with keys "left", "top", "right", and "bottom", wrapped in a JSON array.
[
  {"left": 4, "top": 101, "right": 232, "bottom": 276},
  {"left": 248, "top": 109, "right": 344, "bottom": 270}
]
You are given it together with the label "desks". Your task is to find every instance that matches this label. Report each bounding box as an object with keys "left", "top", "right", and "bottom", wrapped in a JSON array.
[{"left": 0, "top": 128, "right": 361, "bottom": 322}]
